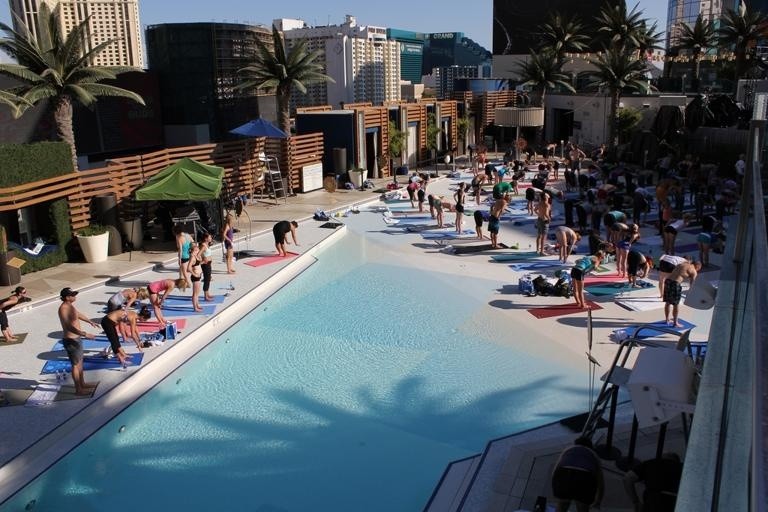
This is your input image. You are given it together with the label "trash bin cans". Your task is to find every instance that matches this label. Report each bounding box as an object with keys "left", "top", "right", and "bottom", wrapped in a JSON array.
[{"left": 272, "top": 173, "right": 289, "bottom": 197}]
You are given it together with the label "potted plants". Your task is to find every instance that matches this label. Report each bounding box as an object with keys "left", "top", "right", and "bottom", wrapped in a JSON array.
[
  {"left": 76, "top": 225, "right": 112, "bottom": 264},
  {"left": 347, "top": 167, "right": 369, "bottom": 187}
]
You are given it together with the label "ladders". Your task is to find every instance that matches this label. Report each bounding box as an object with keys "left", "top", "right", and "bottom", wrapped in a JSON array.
[{"left": 252, "top": 154, "right": 287, "bottom": 207}]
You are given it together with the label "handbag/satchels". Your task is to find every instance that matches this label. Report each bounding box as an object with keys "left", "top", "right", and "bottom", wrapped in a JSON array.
[{"left": 166, "top": 322, "right": 176, "bottom": 339}]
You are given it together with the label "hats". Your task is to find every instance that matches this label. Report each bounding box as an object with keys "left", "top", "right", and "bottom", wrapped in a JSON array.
[{"left": 60, "top": 288, "right": 78, "bottom": 296}]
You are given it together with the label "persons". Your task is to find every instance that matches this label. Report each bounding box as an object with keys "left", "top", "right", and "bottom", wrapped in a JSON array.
[
  {"left": 57, "top": 287, "right": 102, "bottom": 396},
  {"left": 223, "top": 214, "right": 239, "bottom": 274},
  {"left": 407, "top": 136, "right": 748, "bottom": 327},
  {"left": 0, "top": 286, "right": 27, "bottom": 343},
  {"left": 273, "top": 220, "right": 301, "bottom": 257},
  {"left": 101, "top": 224, "right": 213, "bottom": 366}
]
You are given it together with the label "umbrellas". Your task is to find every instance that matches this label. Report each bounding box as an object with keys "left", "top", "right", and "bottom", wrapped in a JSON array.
[{"left": 229, "top": 115, "right": 288, "bottom": 158}]
